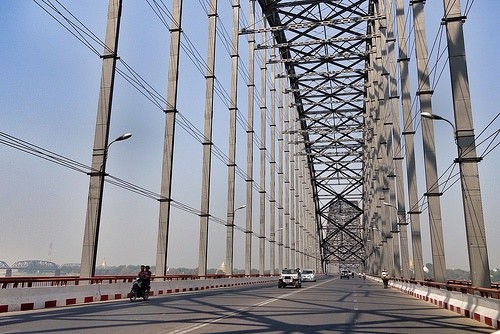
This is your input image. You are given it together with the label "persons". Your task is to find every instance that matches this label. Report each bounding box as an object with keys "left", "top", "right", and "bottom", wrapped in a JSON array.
[
  {"left": 297, "top": 268, "right": 302, "bottom": 275},
  {"left": 358, "top": 271, "right": 361, "bottom": 275},
  {"left": 363, "top": 271, "right": 366, "bottom": 276},
  {"left": 381, "top": 269, "right": 389, "bottom": 287},
  {"left": 137, "top": 265, "right": 152, "bottom": 299}
]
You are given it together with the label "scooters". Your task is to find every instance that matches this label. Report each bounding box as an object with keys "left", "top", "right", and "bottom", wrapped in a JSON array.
[{"left": 129, "top": 278, "right": 149, "bottom": 302}]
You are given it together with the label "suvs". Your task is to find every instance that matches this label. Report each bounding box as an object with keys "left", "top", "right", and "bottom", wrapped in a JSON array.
[
  {"left": 341, "top": 272, "right": 352, "bottom": 279},
  {"left": 277, "top": 268, "right": 302, "bottom": 288}
]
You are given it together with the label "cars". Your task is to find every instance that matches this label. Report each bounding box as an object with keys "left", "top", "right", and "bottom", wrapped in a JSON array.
[{"left": 301, "top": 269, "right": 316, "bottom": 282}]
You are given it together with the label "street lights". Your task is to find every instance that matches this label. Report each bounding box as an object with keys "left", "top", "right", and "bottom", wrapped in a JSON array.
[
  {"left": 77, "top": 133, "right": 134, "bottom": 284},
  {"left": 421, "top": 111, "right": 491, "bottom": 288},
  {"left": 226, "top": 204, "right": 247, "bottom": 277},
  {"left": 269, "top": 228, "right": 284, "bottom": 276},
  {"left": 383, "top": 202, "right": 411, "bottom": 281}
]
